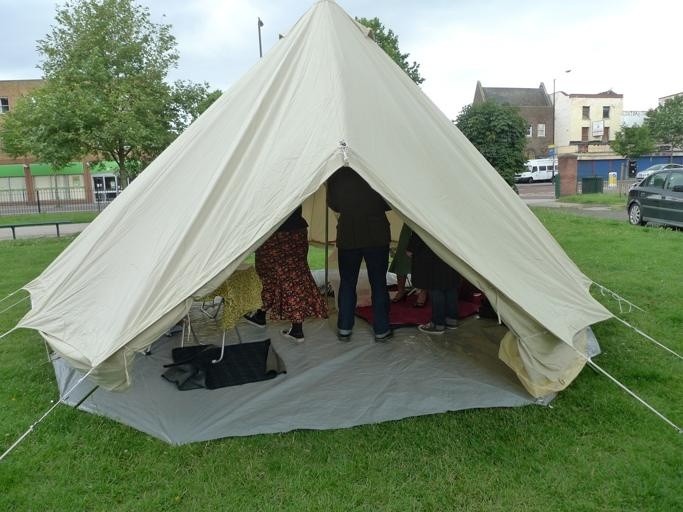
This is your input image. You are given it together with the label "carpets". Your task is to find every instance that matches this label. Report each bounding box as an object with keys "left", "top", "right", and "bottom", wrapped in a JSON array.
[
  {"left": 161, "top": 339, "right": 287, "bottom": 392},
  {"left": 351, "top": 292, "right": 485, "bottom": 332}
]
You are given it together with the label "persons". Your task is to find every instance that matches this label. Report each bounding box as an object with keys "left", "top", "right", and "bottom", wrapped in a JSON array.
[
  {"left": 242, "top": 203, "right": 330, "bottom": 342},
  {"left": 407, "top": 228, "right": 461, "bottom": 335},
  {"left": 326, "top": 166, "right": 394, "bottom": 343},
  {"left": 389, "top": 222, "right": 429, "bottom": 307}
]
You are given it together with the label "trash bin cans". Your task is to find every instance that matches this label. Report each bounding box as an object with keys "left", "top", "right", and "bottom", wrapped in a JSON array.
[
  {"left": 583, "top": 176, "right": 603, "bottom": 195},
  {"left": 608, "top": 172, "right": 618, "bottom": 188}
]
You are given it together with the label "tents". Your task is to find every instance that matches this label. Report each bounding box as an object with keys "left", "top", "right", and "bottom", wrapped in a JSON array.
[{"left": 18, "top": 0, "right": 612, "bottom": 446}]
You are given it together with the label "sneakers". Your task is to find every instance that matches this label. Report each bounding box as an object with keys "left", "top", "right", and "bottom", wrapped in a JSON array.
[
  {"left": 392, "top": 291, "right": 407, "bottom": 303},
  {"left": 417, "top": 323, "right": 459, "bottom": 334},
  {"left": 242, "top": 309, "right": 265, "bottom": 328},
  {"left": 338, "top": 332, "right": 351, "bottom": 342},
  {"left": 414, "top": 293, "right": 428, "bottom": 307},
  {"left": 374, "top": 330, "right": 393, "bottom": 343},
  {"left": 279, "top": 322, "right": 305, "bottom": 343}
]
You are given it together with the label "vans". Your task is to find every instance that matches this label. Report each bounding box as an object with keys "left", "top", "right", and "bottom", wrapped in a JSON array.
[{"left": 514, "top": 159, "right": 556, "bottom": 183}]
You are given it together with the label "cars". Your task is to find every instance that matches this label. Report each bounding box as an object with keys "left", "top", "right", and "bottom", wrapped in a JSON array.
[{"left": 628, "top": 164, "right": 682, "bottom": 230}]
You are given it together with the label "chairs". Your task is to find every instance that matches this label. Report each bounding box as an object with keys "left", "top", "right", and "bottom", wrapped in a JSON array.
[
  {"left": 653, "top": 175, "right": 670, "bottom": 189},
  {"left": 179, "top": 268, "right": 263, "bottom": 365}
]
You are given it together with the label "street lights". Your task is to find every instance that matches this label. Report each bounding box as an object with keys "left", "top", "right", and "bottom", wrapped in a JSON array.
[
  {"left": 552, "top": 69, "right": 571, "bottom": 144},
  {"left": 257, "top": 19, "right": 263, "bottom": 59}
]
